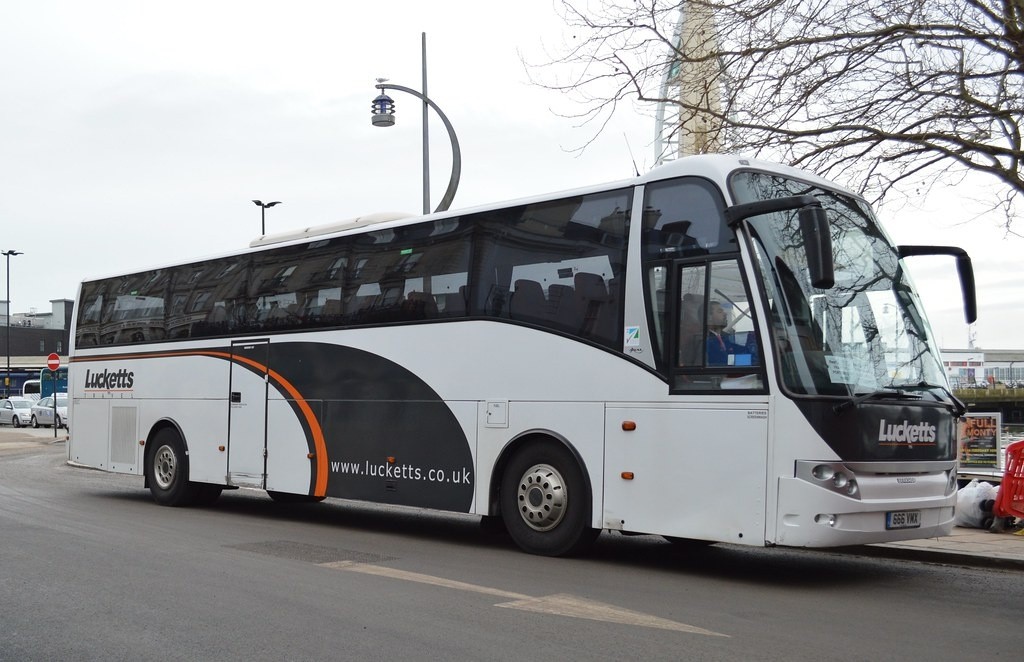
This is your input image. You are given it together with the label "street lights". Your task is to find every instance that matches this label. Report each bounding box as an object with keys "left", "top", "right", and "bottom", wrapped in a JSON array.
[
  {"left": 373, "top": 76, "right": 461, "bottom": 215},
  {"left": 252, "top": 200, "right": 282, "bottom": 235},
  {"left": 0, "top": 249, "right": 25, "bottom": 400}
]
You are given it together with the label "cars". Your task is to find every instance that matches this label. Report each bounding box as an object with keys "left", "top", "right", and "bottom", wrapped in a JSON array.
[
  {"left": 29, "top": 392, "right": 68, "bottom": 429},
  {"left": 0, "top": 399, "right": 32, "bottom": 429}
]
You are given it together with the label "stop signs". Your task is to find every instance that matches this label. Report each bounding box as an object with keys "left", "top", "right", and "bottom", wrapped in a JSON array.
[{"left": 46, "top": 353, "right": 60, "bottom": 371}]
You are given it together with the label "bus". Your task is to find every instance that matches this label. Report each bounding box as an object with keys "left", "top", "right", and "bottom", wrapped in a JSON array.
[
  {"left": 63, "top": 149, "right": 980, "bottom": 558},
  {"left": 0, "top": 367, "right": 67, "bottom": 401}
]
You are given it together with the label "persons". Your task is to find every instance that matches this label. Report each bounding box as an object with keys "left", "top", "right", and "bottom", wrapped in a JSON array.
[{"left": 698, "top": 301, "right": 745, "bottom": 366}]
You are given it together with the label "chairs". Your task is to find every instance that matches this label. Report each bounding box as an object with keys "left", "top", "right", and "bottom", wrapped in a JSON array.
[{"left": 77, "top": 272, "right": 713, "bottom": 369}]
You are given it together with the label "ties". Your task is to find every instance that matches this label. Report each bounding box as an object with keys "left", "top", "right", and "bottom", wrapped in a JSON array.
[{"left": 718, "top": 335, "right": 725, "bottom": 351}]
